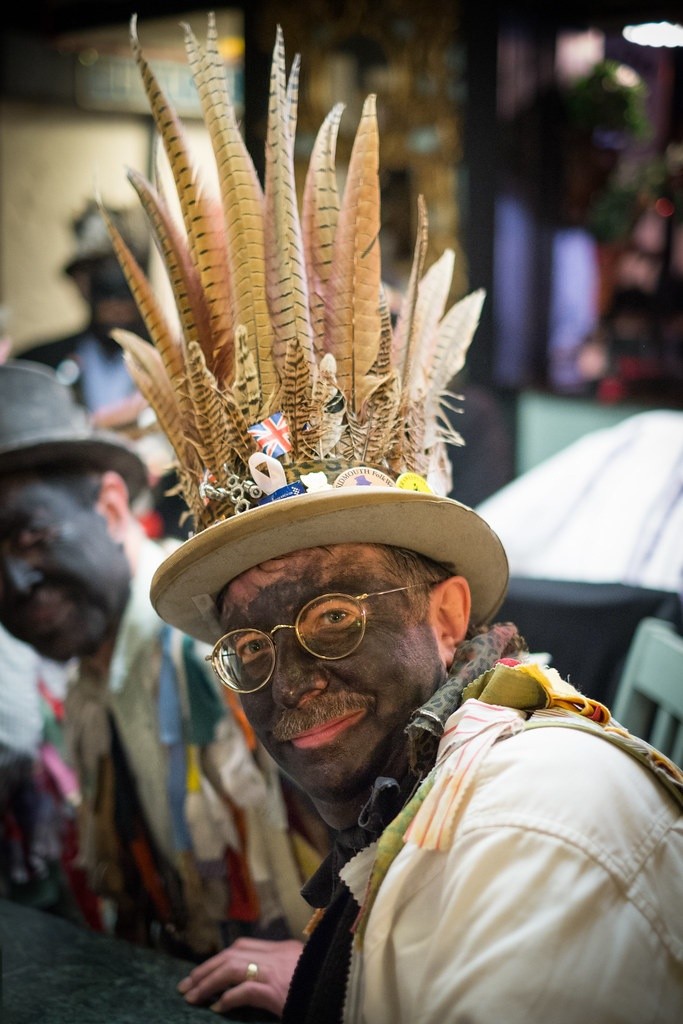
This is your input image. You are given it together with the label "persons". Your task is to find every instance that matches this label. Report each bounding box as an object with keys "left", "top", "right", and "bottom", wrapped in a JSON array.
[
  {"left": 19, "top": 203, "right": 153, "bottom": 413},
  {"left": 151, "top": 488, "right": 683, "bottom": 1024},
  {"left": 0, "top": 366, "right": 338, "bottom": 961}
]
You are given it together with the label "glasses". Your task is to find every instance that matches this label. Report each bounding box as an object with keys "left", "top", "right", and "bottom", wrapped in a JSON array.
[{"left": 204, "top": 581, "right": 441, "bottom": 693}]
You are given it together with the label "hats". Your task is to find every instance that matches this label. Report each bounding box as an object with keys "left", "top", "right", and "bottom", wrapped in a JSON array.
[
  {"left": 0, "top": 360, "right": 149, "bottom": 505},
  {"left": 103, "top": 10, "right": 510, "bottom": 650},
  {"left": 65, "top": 208, "right": 147, "bottom": 272}
]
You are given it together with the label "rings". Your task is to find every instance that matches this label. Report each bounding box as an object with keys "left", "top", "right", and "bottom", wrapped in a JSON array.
[{"left": 245, "top": 963, "right": 257, "bottom": 980}]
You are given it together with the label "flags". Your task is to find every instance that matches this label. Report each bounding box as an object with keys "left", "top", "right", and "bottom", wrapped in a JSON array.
[{"left": 249, "top": 411, "right": 310, "bottom": 459}]
